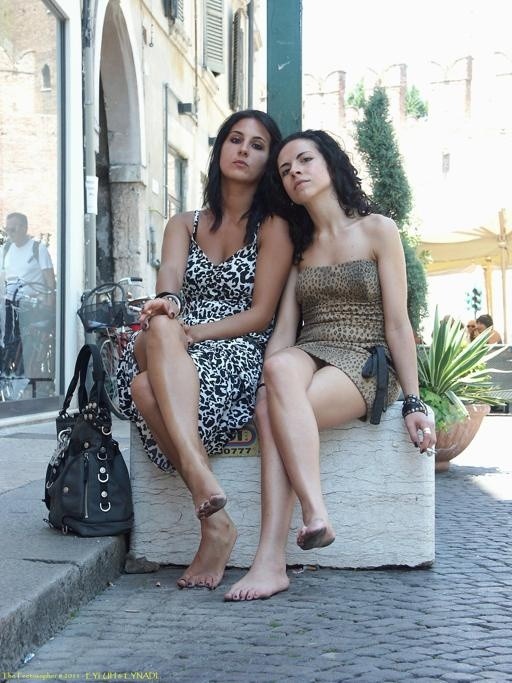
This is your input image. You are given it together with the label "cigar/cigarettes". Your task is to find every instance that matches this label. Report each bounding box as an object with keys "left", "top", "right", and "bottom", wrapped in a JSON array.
[{"left": 418, "top": 444, "right": 437, "bottom": 454}]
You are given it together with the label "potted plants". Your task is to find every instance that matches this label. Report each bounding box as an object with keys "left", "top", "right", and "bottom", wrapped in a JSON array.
[{"left": 398, "top": 299, "right": 512, "bottom": 473}]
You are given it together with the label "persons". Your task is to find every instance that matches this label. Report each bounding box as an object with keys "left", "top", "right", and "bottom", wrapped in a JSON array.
[
  {"left": 431, "top": 314, "right": 462, "bottom": 339},
  {"left": 224, "top": 129, "right": 436, "bottom": 601},
  {"left": 463, "top": 319, "right": 478, "bottom": 342},
  {"left": 477, "top": 314, "right": 502, "bottom": 344},
  {"left": 1, "top": 212, "right": 56, "bottom": 375},
  {"left": 115, "top": 109, "right": 297, "bottom": 588}
]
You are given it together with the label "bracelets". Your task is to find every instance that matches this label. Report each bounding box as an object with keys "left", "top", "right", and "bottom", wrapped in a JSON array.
[
  {"left": 154, "top": 290, "right": 185, "bottom": 316},
  {"left": 402, "top": 394, "right": 426, "bottom": 417}
]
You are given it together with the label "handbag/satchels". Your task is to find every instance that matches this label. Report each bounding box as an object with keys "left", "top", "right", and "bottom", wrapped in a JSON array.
[{"left": 45, "top": 343, "right": 134, "bottom": 537}]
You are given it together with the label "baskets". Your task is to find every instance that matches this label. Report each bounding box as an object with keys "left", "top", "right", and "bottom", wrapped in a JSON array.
[{"left": 77, "top": 300, "right": 125, "bottom": 332}]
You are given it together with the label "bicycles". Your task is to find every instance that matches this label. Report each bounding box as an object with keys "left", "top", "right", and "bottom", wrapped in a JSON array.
[
  {"left": 1, "top": 277, "right": 60, "bottom": 403},
  {"left": 80, "top": 274, "right": 153, "bottom": 421}
]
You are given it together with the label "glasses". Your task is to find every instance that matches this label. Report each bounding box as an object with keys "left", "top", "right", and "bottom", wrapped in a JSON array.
[{"left": 467, "top": 325, "right": 475, "bottom": 328}]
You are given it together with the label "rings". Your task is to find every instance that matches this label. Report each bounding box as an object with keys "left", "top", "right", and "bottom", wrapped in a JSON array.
[{"left": 422, "top": 427, "right": 431, "bottom": 434}]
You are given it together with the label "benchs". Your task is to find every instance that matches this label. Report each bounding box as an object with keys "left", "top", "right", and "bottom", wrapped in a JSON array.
[{"left": 122, "top": 396, "right": 436, "bottom": 572}]
